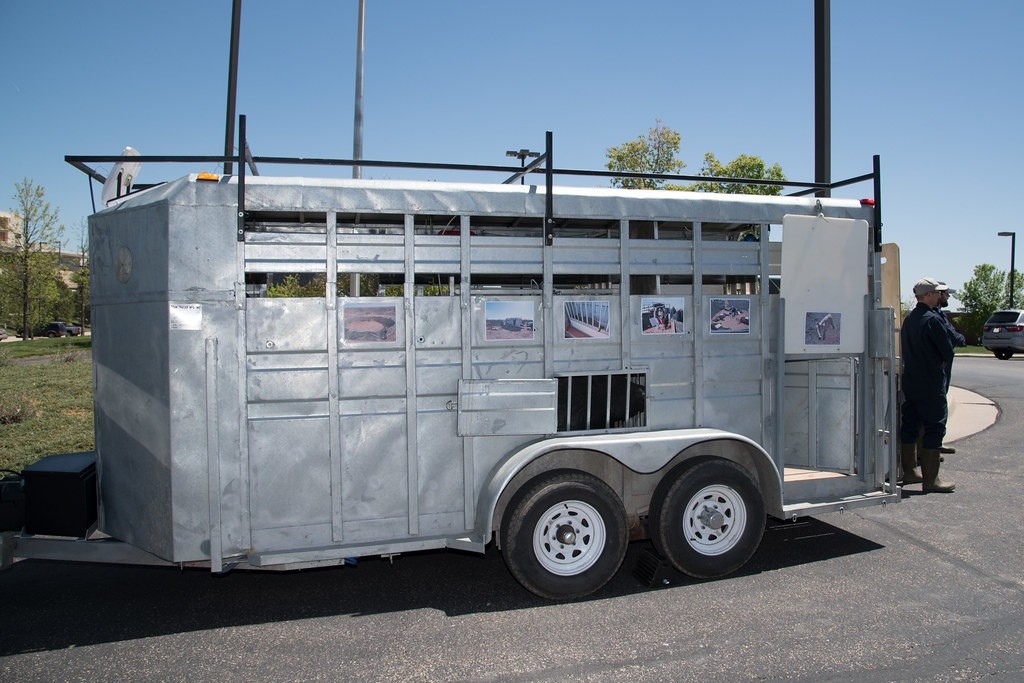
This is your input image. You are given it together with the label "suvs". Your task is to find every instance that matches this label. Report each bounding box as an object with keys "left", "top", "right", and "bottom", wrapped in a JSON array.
[{"left": 982, "top": 309, "right": 1024, "bottom": 360}]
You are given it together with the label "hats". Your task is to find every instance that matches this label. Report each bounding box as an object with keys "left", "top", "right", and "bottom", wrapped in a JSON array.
[
  {"left": 913, "top": 278, "right": 949, "bottom": 295},
  {"left": 937, "top": 281, "right": 956, "bottom": 293}
]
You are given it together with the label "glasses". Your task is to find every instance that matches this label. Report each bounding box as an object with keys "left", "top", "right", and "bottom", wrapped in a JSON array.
[{"left": 930, "top": 290, "right": 942, "bottom": 296}]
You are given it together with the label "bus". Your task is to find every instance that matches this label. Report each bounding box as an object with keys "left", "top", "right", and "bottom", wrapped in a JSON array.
[{"left": 87, "top": 173, "right": 905, "bottom": 601}]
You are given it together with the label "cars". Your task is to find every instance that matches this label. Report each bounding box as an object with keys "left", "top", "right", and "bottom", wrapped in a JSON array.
[{"left": 15, "top": 321, "right": 81, "bottom": 338}]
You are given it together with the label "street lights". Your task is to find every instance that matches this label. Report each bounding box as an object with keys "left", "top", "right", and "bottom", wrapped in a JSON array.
[
  {"left": 997, "top": 232, "right": 1016, "bottom": 309},
  {"left": 39, "top": 242, "right": 46, "bottom": 321},
  {"left": 54, "top": 240, "right": 61, "bottom": 265},
  {"left": 504, "top": 148, "right": 541, "bottom": 296}
]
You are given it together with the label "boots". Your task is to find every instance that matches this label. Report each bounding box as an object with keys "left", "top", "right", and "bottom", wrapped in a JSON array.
[
  {"left": 922, "top": 448, "right": 955, "bottom": 492},
  {"left": 900, "top": 442, "right": 923, "bottom": 483}
]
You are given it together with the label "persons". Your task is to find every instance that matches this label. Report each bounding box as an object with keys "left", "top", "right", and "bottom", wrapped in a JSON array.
[
  {"left": 729, "top": 305, "right": 737, "bottom": 316},
  {"left": 886, "top": 277, "right": 965, "bottom": 490}
]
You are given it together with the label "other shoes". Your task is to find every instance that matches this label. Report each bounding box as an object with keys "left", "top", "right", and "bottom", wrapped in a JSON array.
[
  {"left": 917, "top": 455, "right": 944, "bottom": 466},
  {"left": 941, "top": 446, "right": 956, "bottom": 454}
]
